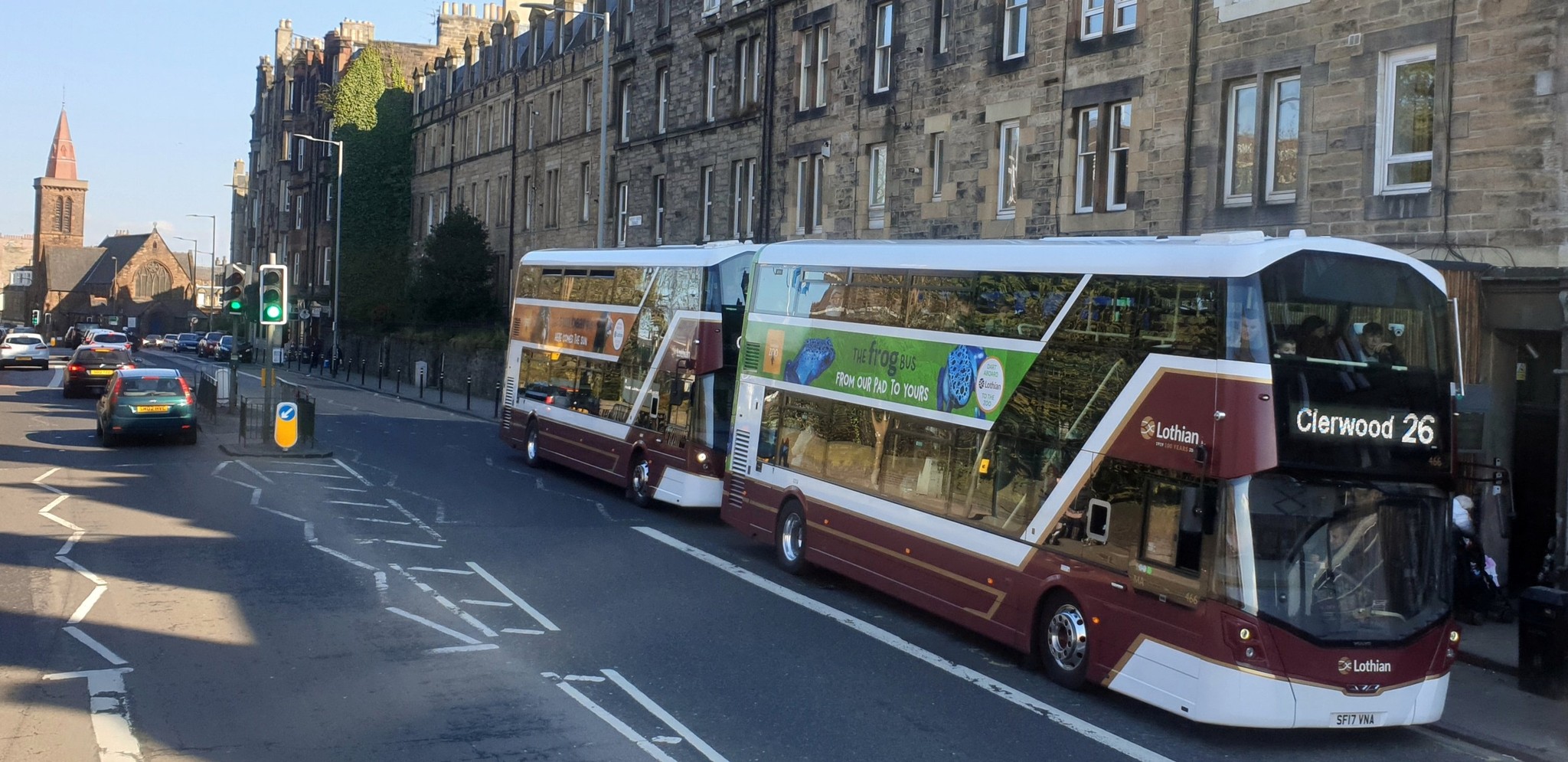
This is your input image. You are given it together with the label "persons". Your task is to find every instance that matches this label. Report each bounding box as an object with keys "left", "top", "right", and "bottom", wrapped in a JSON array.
[
  {"left": 1223, "top": 516, "right": 1375, "bottom": 609},
  {"left": 1450, "top": 495, "right": 1497, "bottom": 624},
  {"left": 593, "top": 310, "right": 613, "bottom": 354},
  {"left": 825, "top": 289, "right": 855, "bottom": 318},
  {"left": 791, "top": 414, "right": 823, "bottom": 467},
  {"left": 1232, "top": 309, "right": 1411, "bottom": 404},
  {"left": 311, "top": 335, "right": 343, "bottom": 378},
  {"left": 679, "top": 369, "right": 697, "bottom": 408}
]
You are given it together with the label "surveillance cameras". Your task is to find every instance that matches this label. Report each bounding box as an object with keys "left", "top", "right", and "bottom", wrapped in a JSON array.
[
  {"left": 1394, "top": 326, "right": 1404, "bottom": 337},
  {"left": 1524, "top": 343, "right": 1540, "bottom": 359}
]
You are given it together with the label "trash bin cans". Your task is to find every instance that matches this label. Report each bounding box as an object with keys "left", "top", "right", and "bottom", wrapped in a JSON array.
[
  {"left": 1518, "top": 587, "right": 1567, "bottom": 699},
  {"left": 302, "top": 347, "right": 312, "bottom": 363}
]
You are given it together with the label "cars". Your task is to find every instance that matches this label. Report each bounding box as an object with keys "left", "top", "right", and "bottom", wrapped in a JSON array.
[
  {"left": 214, "top": 335, "right": 252, "bottom": 362},
  {"left": 0, "top": 333, "right": 50, "bottom": 370},
  {"left": 13, "top": 327, "right": 39, "bottom": 333},
  {"left": 61, "top": 344, "right": 198, "bottom": 445},
  {"left": 143, "top": 332, "right": 224, "bottom": 358}
]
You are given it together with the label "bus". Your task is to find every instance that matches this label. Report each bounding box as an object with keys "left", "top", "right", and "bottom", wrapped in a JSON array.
[
  {"left": 499, "top": 238, "right": 762, "bottom": 508},
  {"left": 721, "top": 228, "right": 1480, "bottom": 729}
]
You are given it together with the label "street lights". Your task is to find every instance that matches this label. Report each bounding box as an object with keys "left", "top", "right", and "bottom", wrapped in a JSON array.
[
  {"left": 187, "top": 214, "right": 216, "bottom": 332},
  {"left": 225, "top": 184, "right": 260, "bottom": 343},
  {"left": 520, "top": 3, "right": 609, "bottom": 249},
  {"left": 293, "top": 134, "right": 342, "bottom": 370},
  {"left": 112, "top": 256, "right": 117, "bottom": 314},
  {"left": 172, "top": 237, "right": 197, "bottom": 333}
]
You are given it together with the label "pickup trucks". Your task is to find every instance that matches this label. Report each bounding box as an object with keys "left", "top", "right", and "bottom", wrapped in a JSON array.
[{"left": 525, "top": 382, "right": 572, "bottom": 407}]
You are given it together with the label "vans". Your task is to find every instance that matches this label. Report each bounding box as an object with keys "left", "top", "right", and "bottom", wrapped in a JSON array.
[{"left": 72, "top": 323, "right": 101, "bottom": 350}]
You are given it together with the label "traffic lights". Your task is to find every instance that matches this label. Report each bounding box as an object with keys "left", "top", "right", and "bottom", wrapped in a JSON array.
[
  {"left": 222, "top": 264, "right": 253, "bottom": 315},
  {"left": 259, "top": 264, "right": 287, "bottom": 325},
  {"left": 32, "top": 310, "right": 39, "bottom": 325}
]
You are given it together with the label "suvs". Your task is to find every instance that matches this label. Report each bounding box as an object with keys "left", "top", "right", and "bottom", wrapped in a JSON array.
[
  {"left": 81, "top": 329, "right": 132, "bottom": 358},
  {"left": 65, "top": 326, "right": 74, "bottom": 348}
]
